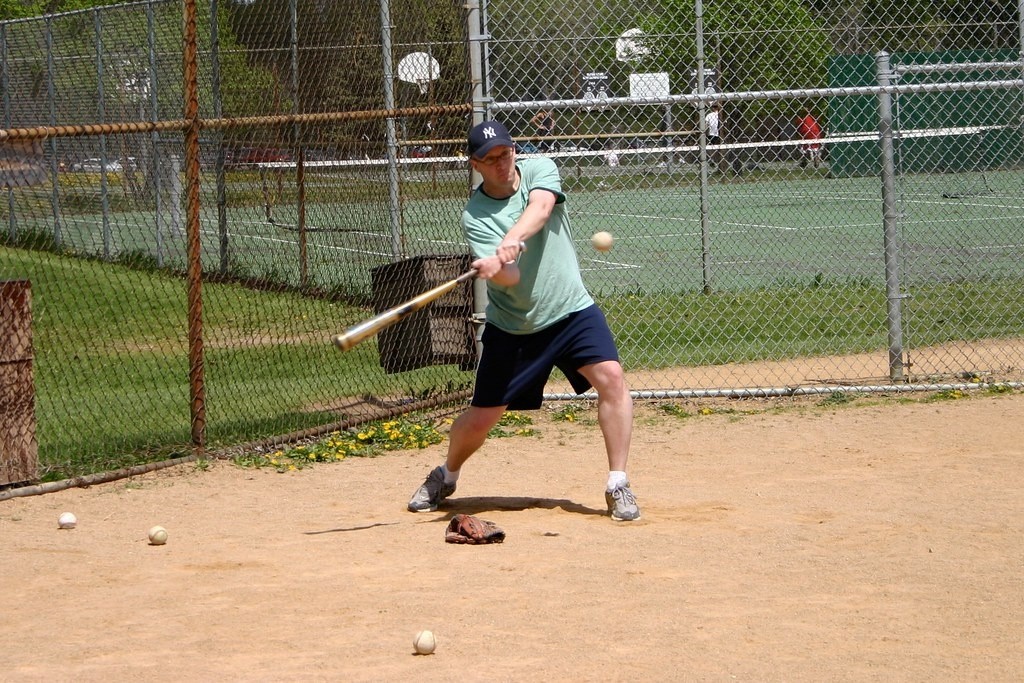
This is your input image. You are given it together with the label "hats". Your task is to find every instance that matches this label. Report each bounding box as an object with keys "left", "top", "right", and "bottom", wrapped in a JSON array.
[{"left": 468, "top": 121, "right": 514, "bottom": 159}]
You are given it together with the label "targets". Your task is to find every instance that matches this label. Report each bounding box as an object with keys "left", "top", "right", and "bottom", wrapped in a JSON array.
[
  {"left": 691, "top": 69, "right": 717, "bottom": 107},
  {"left": 582, "top": 74, "right": 610, "bottom": 118}
]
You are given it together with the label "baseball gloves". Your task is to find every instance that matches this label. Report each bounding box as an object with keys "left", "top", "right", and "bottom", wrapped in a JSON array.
[{"left": 445, "top": 514, "right": 507, "bottom": 544}]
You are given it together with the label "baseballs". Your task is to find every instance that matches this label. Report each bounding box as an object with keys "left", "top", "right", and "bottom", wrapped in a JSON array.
[
  {"left": 412, "top": 629, "right": 439, "bottom": 655},
  {"left": 592, "top": 231, "right": 614, "bottom": 252},
  {"left": 147, "top": 525, "right": 169, "bottom": 544},
  {"left": 57, "top": 511, "right": 78, "bottom": 529}
]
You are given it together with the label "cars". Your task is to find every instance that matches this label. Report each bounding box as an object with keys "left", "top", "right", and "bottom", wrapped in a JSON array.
[{"left": 66, "top": 141, "right": 366, "bottom": 173}]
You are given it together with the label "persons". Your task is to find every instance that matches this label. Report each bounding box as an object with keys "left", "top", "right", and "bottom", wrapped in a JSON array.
[
  {"left": 704, "top": 100, "right": 722, "bottom": 165},
  {"left": 793, "top": 105, "right": 823, "bottom": 166},
  {"left": 407, "top": 122, "right": 641, "bottom": 522},
  {"left": 532, "top": 108, "right": 555, "bottom": 151}
]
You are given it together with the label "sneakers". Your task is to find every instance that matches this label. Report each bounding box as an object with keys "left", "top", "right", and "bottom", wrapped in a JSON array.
[
  {"left": 408, "top": 466, "right": 456, "bottom": 512},
  {"left": 605, "top": 479, "right": 641, "bottom": 521}
]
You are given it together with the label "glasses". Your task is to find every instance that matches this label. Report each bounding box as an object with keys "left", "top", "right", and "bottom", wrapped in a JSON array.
[{"left": 471, "top": 146, "right": 514, "bottom": 166}]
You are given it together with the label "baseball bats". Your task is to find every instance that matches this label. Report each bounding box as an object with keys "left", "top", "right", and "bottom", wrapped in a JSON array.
[{"left": 333, "top": 241, "right": 528, "bottom": 353}]
